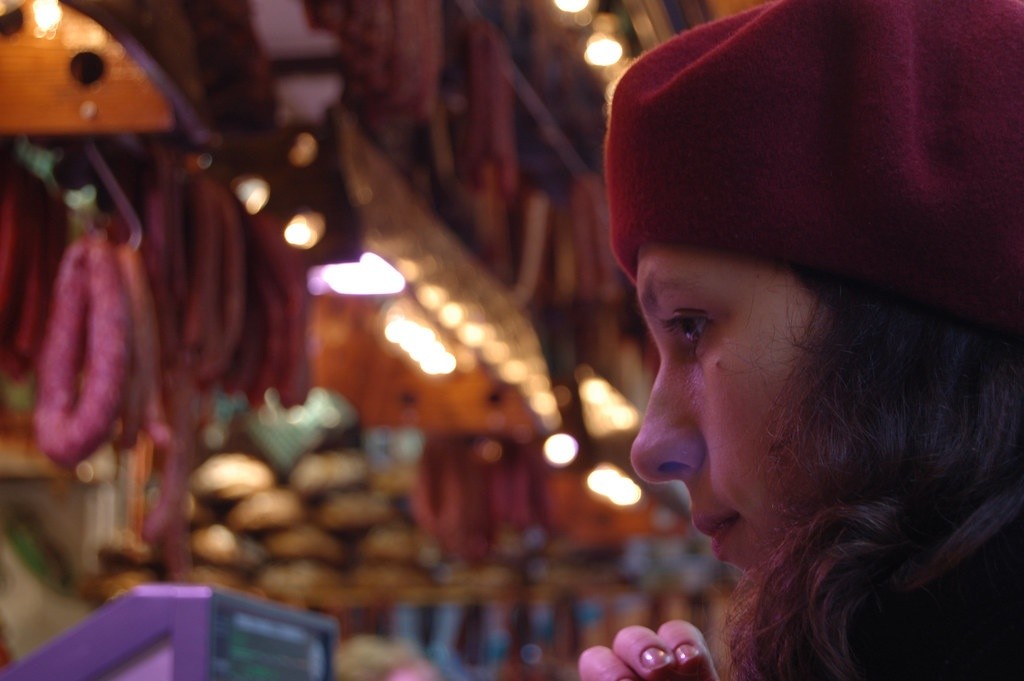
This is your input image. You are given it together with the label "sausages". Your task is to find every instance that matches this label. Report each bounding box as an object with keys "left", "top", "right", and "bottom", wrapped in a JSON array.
[
  {"left": 0, "top": 146, "right": 314, "bottom": 587},
  {"left": 354, "top": 1, "right": 628, "bottom": 305}
]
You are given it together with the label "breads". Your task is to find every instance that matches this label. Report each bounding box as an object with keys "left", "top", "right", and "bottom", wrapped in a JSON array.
[{"left": 88, "top": 451, "right": 521, "bottom": 609}]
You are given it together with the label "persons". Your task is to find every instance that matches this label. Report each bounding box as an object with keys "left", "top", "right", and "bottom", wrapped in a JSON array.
[{"left": 579, "top": 0, "right": 1024, "bottom": 681}]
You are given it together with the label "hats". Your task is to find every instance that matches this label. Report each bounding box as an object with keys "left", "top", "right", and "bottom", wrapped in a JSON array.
[{"left": 604, "top": 0, "right": 1023, "bottom": 331}]
